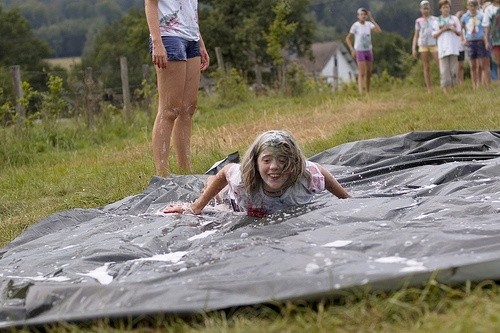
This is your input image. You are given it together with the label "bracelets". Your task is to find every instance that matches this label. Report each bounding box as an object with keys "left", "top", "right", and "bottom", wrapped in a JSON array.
[{"left": 188, "top": 205, "right": 198, "bottom": 214}]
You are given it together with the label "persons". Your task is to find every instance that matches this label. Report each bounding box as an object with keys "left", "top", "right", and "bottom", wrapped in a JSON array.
[
  {"left": 411, "top": 0, "right": 500, "bottom": 95},
  {"left": 145, "top": 0, "right": 210, "bottom": 178},
  {"left": 163, "top": 130, "right": 354, "bottom": 217},
  {"left": 346, "top": 7, "right": 382, "bottom": 98}
]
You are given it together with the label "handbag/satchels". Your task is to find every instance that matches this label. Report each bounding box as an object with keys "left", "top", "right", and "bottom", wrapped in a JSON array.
[{"left": 205, "top": 151, "right": 240, "bottom": 175}]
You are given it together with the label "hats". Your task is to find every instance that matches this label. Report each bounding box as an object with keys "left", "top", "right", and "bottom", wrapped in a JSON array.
[
  {"left": 357, "top": 7, "right": 367, "bottom": 14},
  {"left": 420, "top": 0, "right": 431, "bottom": 8},
  {"left": 466, "top": 0, "right": 478, "bottom": 5}
]
